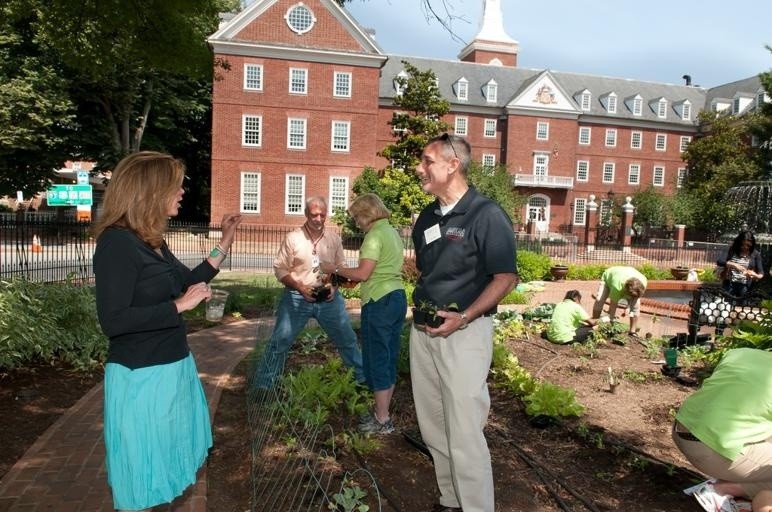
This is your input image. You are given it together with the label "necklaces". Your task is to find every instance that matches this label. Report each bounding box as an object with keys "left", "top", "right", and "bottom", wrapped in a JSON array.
[{"left": 303, "top": 222, "right": 325, "bottom": 257}]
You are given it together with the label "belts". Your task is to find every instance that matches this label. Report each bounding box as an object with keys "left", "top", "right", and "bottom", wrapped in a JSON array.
[{"left": 673, "top": 419, "right": 700, "bottom": 442}]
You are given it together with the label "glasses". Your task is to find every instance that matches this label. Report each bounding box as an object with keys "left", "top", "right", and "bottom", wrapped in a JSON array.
[{"left": 439, "top": 133, "right": 458, "bottom": 158}]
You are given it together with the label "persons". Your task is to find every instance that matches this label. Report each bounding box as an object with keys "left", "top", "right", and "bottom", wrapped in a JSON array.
[
  {"left": 713, "top": 229, "right": 765, "bottom": 340},
  {"left": 670, "top": 345, "right": 772, "bottom": 512},
  {"left": 92, "top": 149, "right": 245, "bottom": 510},
  {"left": 546, "top": 288, "right": 612, "bottom": 346},
  {"left": 408, "top": 131, "right": 520, "bottom": 512},
  {"left": 590, "top": 265, "right": 649, "bottom": 338},
  {"left": 247, "top": 195, "right": 370, "bottom": 404},
  {"left": 318, "top": 193, "right": 408, "bottom": 435}
]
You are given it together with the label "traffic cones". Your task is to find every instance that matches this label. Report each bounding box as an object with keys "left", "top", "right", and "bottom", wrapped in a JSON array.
[{"left": 32, "top": 234, "right": 42, "bottom": 253}]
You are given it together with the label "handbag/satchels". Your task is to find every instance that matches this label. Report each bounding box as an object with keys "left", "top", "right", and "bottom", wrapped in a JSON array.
[{"left": 712, "top": 264, "right": 733, "bottom": 282}]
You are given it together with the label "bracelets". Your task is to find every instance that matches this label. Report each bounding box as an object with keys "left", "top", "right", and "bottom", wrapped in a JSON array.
[
  {"left": 459, "top": 310, "right": 469, "bottom": 332},
  {"left": 210, "top": 243, "right": 229, "bottom": 265},
  {"left": 335, "top": 265, "right": 340, "bottom": 278}
]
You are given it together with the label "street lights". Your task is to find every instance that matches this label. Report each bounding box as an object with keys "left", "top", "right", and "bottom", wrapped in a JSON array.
[
  {"left": 529, "top": 209, "right": 535, "bottom": 234},
  {"left": 740, "top": 221, "right": 748, "bottom": 232},
  {"left": 568, "top": 202, "right": 574, "bottom": 233}
]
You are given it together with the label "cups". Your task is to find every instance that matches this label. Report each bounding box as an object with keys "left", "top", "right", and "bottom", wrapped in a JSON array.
[{"left": 205, "top": 289, "right": 230, "bottom": 323}]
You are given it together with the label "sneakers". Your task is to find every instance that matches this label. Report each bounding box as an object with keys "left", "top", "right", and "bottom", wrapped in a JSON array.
[
  {"left": 357, "top": 411, "right": 395, "bottom": 435},
  {"left": 693, "top": 480, "right": 738, "bottom": 512},
  {"left": 629, "top": 333, "right": 639, "bottom": 338}
]
